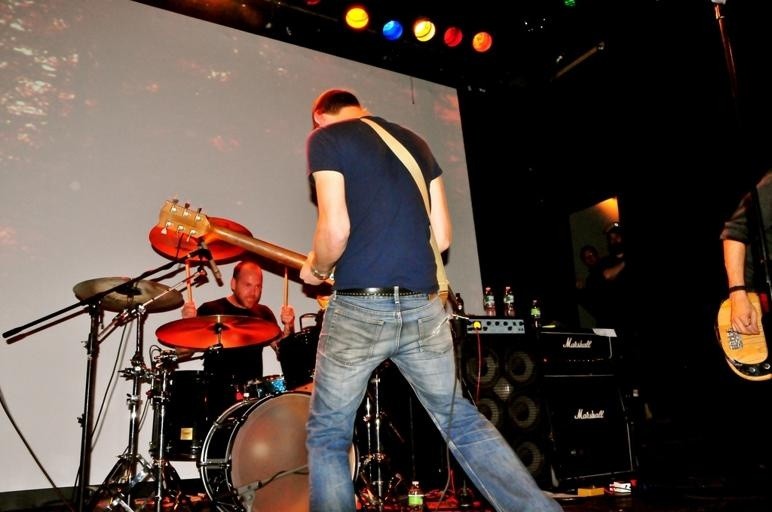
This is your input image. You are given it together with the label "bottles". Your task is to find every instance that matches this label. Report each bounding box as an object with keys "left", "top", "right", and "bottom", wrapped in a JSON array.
[
  {"left": 455, "top": 287, "right": 541, "bottom": 328},
  {"left": 407, "top": 481, "right": 424, "bottom": 512}
]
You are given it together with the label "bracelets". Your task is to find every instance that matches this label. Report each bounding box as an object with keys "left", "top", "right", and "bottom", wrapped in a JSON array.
[
  {"left": 310, "top": 267, "right": 332, "bottom": 280},
  {"left": 727, "top": 286, "right": 747, "bottom": 293}
]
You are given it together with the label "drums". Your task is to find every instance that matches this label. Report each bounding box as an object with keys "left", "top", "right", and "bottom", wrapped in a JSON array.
[
  {"left": 199, "top": 392, "right": 361, "bottom": 511},
  {"left": 277, "top": 327, "right": 322, "bottom": 391},
  {"left": 149, "top": 370, "right": 230, "bottom": 462}
]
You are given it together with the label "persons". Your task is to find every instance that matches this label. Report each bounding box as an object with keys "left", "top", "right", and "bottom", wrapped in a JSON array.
[
  {"left": 176, "top": 259, "right": 295, "bottom": 380},
  {"left": 300, "top": 90, "right": 567, "bottom": 512},
  {"left": 566, "top": 217, "right": 628, "bottom": 330},
  {"left": 719, "top": 166, "right": 772, "bottom": 337}
]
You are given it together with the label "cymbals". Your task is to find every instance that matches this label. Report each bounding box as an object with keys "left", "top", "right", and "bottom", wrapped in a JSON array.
[
  {"left": 246, "top": 374, "right": 279, "bottom": 386},
  {"left": 74, "top": 277, "right": 183, "bottom": 311},
  {"left": 150, "top": 216, "right": 254, "bottom": 262},
  {"left": 156, "top": 315, "right": 281, "bottom": 349}
]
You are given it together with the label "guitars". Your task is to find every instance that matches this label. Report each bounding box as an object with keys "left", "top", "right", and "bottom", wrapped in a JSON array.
[
  {"left": 158, "top": 199, "right": 448, "bottom": 308},
  {"left": 713, "top": 287, "right": 772, "bottom": 382}
]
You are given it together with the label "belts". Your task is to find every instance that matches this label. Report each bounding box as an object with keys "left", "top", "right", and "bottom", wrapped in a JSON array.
[{"left": 336, "top": 287, "right": 418, "bottom": 296}]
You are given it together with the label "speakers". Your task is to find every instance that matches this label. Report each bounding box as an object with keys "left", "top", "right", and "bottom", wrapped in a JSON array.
[{"left": 410, "top": 332, "right": 552, "bottom": 500}]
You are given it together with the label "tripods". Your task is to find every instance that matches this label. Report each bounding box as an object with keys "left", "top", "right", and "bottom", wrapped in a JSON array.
[
  {"left": 100, "top": 390, "right": 196, "bottom": 512},
  {"left": 87, "top": 356, "right": 187, "bottom": 512},
  {"left": 359, "top": 370, "right": 430, "bottom": 512}
]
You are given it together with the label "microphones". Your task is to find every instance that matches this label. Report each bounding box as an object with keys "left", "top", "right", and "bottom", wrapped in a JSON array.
[{"left": 201, "top": 238, "right": 225, "bottom": 287}]
